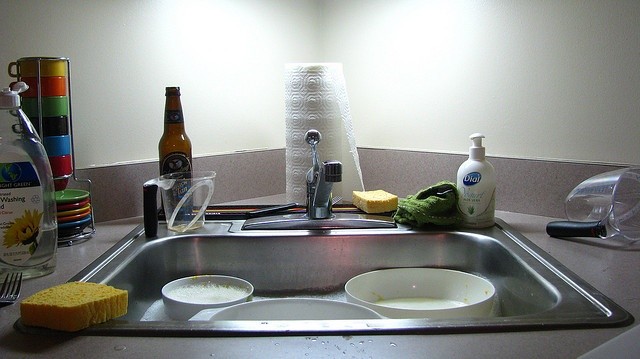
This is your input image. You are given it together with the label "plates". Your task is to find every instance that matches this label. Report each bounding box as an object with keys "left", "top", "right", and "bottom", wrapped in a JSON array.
[{"left": 45, "top": 189, "right": 91, "bottom": 237}]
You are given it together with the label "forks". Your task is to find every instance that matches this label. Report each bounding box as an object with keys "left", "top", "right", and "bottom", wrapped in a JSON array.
[{"left": 0, "top": 272, "right": 22, "bottom": 307}]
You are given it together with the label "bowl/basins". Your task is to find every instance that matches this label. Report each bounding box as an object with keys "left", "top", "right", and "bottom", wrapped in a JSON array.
[
  {"left": 344, "top": 268, "right": 496, "bottom": 319},
  {"left": 160, "top": 275, "right": 254, "bottom": 320},
  {"left": 188, "top": 297, "right": 383, "bottom": 321}
]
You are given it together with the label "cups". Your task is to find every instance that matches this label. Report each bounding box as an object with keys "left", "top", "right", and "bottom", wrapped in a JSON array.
[
  {"left": 7, "top": 57, "right": 68, "bottom": 78},
  {"left": 143, "top": 170, "right": 217, "bottom": 238},
  {"left": 10, "top": 73, "right": 68, "bottom": 97},
  {"left": 545, "top": 164, "right": 640, "bottom": 246},
  {"left": 44, "top": 154, "right": 72, "bottom": 190},
  {"left": 15, "top": 136, "right": 70, "bottom": 157},
  {"left": 9, "top": 93, "right": 69, "bottom": 118},
  {"left": 12, "top": 115, "right": 68, "bottom": 136}
]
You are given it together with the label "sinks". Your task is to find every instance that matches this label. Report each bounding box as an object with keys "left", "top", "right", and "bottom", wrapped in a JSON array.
[{"left": 98, "top": 232, "right": 560, "bottom": 321}]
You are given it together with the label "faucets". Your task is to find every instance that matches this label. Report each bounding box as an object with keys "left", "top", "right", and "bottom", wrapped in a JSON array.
[{"left": 304, "top": 130, "right": 344, "bottom": 220}]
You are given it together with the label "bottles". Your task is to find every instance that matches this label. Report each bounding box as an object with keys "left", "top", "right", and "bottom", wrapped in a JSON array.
[{"left": 160, "top": 86, "right": 192, "bottom": 218}]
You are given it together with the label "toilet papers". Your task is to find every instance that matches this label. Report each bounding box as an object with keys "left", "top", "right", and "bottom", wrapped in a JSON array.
[{"left": 284, "top": 63, "right": 364, "bottom": 206}]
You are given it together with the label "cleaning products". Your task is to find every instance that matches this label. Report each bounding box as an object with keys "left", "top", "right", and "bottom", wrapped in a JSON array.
[{"left": 456, "top": 132, "right": 496, "bottom": 228}]
